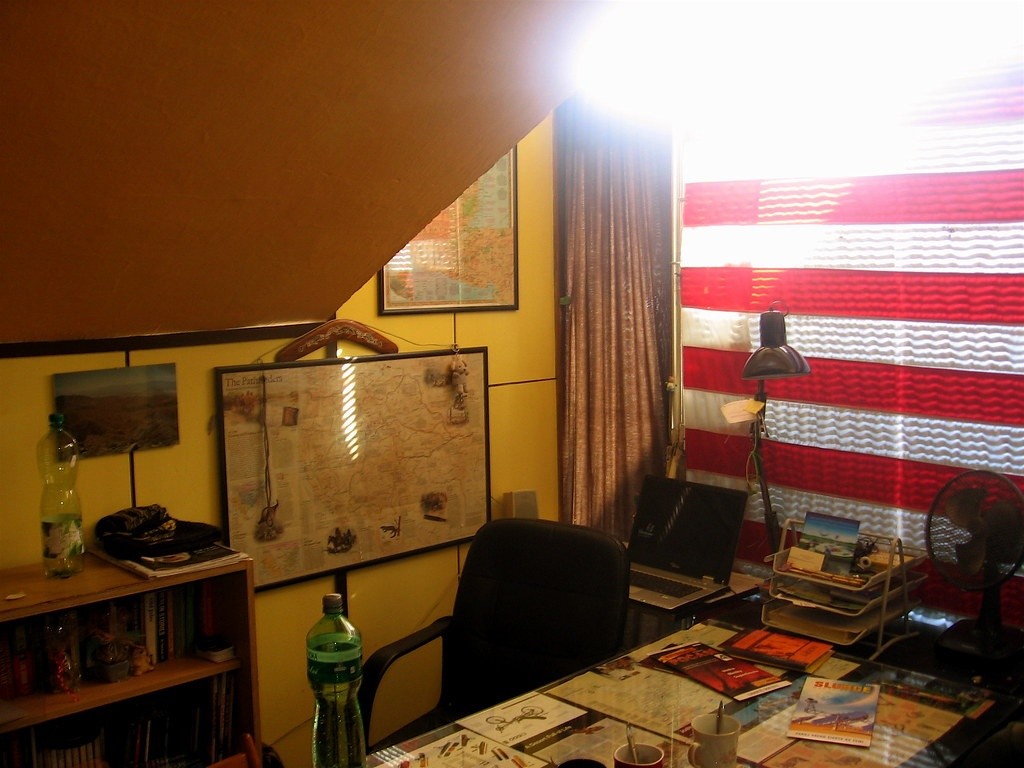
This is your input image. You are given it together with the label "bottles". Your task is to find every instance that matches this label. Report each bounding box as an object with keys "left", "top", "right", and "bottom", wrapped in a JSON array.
[
  {"left": 306, "top": 593, "right": 366, "bottom": 768},
  {"left": 36, "top": 412, "right": 86, "bottom": 577}
]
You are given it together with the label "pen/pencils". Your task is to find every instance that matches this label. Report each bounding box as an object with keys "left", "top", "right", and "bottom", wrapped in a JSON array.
[{"left": 789, "top": 566, "right": 833, "bottom": 581}]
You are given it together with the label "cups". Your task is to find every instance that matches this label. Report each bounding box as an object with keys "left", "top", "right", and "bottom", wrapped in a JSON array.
[
  {"left": 557, "top": 758, "right": 607, "bottom": 768},
  {"left": 614, "top": 742, "right": 664, "bottom": 768},
  {"left": 688, "top": 713, "right": 742, "bottom": 768}
]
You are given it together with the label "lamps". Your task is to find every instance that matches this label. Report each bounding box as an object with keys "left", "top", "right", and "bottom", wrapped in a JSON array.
[{"left": 741, "top": 310, "right": 812, "bottom": 558}]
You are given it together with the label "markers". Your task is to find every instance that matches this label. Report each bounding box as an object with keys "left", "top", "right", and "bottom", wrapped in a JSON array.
[{"left": 832, "top": 575, "right": 866, "bottom": 588}]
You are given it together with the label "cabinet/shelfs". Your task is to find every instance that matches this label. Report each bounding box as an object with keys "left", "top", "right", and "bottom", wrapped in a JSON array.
[
  {"left": 758, "top": 516, "right": 928, "bottom": 662},
  {"left": 0, "top": 547, "right": 262, "bottom": 768}
]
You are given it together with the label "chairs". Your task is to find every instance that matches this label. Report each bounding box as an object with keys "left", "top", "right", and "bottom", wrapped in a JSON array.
[{"left": 357, "top": 518, "right": 631, "bottom": 756}]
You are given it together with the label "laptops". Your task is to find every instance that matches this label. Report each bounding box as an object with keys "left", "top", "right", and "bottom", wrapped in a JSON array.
[{"left": 626, "top": 474, "right": 748, "bottom": 612}]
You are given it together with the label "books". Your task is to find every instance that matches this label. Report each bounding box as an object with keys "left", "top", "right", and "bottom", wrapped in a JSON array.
[
  {"left": 87, "top": 541, "right": 250, "bottom": 578},
  {"left": 786, "top": 677, "right": 880, "bottom": 748},
  {"left": 125, "top": 577, "right": 213, "bottom": 664},
  {"left": 717, "top": 628, "right": 835, "bottom": 674},
  {"left": 0, "top": 672, "right": 235, "bottom": 768}
]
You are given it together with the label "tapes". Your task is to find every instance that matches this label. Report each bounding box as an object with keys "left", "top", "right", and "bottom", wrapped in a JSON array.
[{"left": 859, "top": 557, "right": 871, "bottom": 568}]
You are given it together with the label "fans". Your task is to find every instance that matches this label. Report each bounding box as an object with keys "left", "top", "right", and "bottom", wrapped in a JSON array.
[{"left": 926, "top": 469, "right": 1024, "bottom": 674}]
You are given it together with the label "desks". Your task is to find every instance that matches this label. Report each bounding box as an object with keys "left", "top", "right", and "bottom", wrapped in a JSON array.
[
  {"left": 626, "top": 577, "right": 764, "bottom": 650},
  {"left": 366, "top": 601, "right": 1024, "bottom": 768}
]
